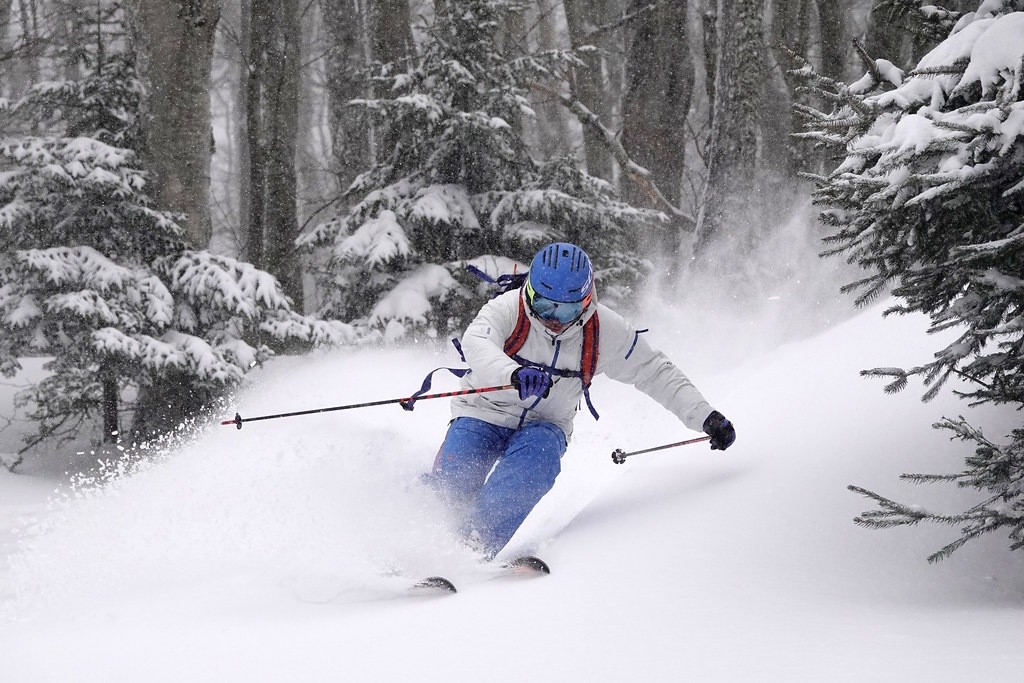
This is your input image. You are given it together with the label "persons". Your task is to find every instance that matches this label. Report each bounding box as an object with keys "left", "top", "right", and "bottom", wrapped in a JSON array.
[{"left": 422, "top": 241, "right": 736, "bottom": 564}]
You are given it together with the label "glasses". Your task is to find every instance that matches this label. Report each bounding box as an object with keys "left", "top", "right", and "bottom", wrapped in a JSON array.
[{"left": 531, "top": 293, "right": 583, "bottom": 325}]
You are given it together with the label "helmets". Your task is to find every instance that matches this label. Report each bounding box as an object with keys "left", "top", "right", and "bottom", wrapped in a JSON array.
[{"left": 525, "top": 242, "right": 594, "bottom": 324}]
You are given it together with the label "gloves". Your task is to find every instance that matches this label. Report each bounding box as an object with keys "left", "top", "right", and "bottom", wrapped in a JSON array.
[
  {"left": 702, "top": 410, "right": 736, "bottom": 452},
  {"left": 510, "top": 366, "right": 551, "bottom": 401}
]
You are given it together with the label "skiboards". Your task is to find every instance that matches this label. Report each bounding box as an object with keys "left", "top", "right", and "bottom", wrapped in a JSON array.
[{"left": 413, "top": 555, "right": 550, "bottom": 596}]
